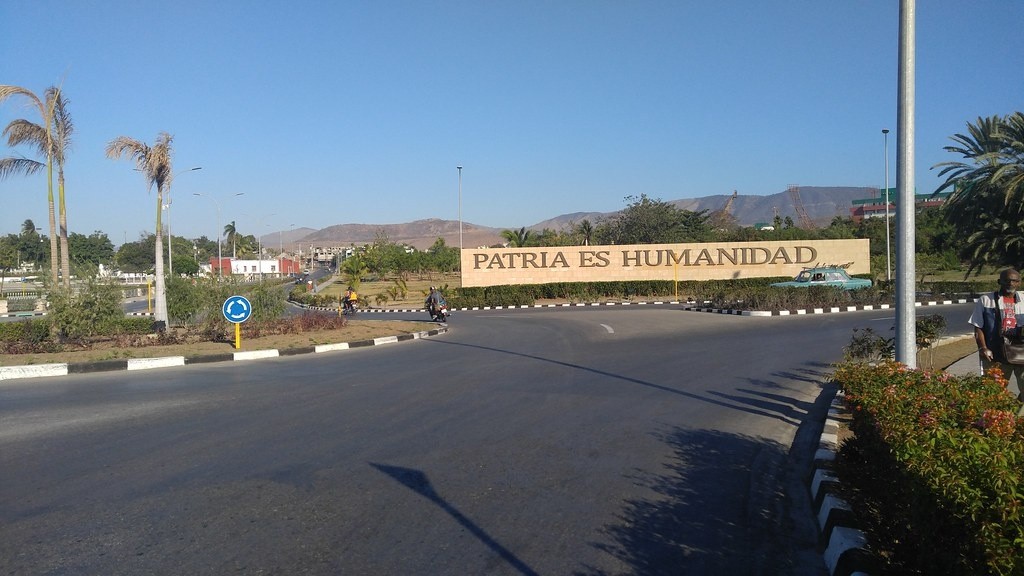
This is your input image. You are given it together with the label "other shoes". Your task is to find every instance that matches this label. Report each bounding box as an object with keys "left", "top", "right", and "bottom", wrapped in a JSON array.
[
  {"left": 344, "top": 309, "right": 349, "bottom": 311},
  {"left": 343, "top": 310, "right": 347, "bottom": 312},
  {"left": 432, "top": 315, "right": 437, "bottom": 319}
]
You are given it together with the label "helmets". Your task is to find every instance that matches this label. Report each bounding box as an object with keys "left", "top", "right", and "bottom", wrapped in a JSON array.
[
  {"left": 348, "top": 287, "right": 352, "bottom": 290},
  {"left": 430, "top": 286, "right": 438, "bottom": 293}
]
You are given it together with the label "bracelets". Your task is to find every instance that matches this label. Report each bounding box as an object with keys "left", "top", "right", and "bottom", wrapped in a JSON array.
[{"left": 978, "top": 347, "right": 987, "bottom": 350}]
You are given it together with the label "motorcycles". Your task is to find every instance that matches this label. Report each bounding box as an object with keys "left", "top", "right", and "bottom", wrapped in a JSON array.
[
  {"left": 344, "top": 301, "right": 357, "bottom": 316},
  {"left": 426, "top": 302, "right": 452, "bottom": 322}
]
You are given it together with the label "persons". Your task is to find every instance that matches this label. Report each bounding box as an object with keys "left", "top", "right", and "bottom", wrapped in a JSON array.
[
  {"left": 341, "top": 286, "right": 357, "bottom": 311},
  {"left": 967, "top": 268, "right": 1024, "bottom": 405},
  {"left": 424, "top": 285, "right": 445, "bottom": 319},
  {"left": 815, "top": 274, "right": 824, "bottom": 281}
]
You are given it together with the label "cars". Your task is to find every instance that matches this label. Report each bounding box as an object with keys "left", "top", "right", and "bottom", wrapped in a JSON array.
[{"left": 771, "top": 268, "right": 872, "bottom": 291}]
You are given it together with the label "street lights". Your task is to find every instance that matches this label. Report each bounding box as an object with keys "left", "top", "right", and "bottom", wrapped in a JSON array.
[
  {"left": 194, "top": 192, "right": 244, "bottom": 285},
  {"left": 881, "top": 128, "right": 890, "bottom": 281},
  {"left": 456, "top": 166, "right": 463, "bottom": 251},
  {"left": 258, "top": 213, "right": 295, "bottom": 288},
  {"left": 132, "top": 167, "right": 202, "bottom": 283}
]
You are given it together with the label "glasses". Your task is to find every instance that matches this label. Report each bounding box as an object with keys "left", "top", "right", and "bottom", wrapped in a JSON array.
[{"left": 1000, "top": 278, "right": 1020, "bottom": 284}]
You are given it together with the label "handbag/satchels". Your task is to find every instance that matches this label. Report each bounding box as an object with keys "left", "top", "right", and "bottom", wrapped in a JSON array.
[{"left": 1000, "top": 335, "right": 1024, "bottom": 365}]
